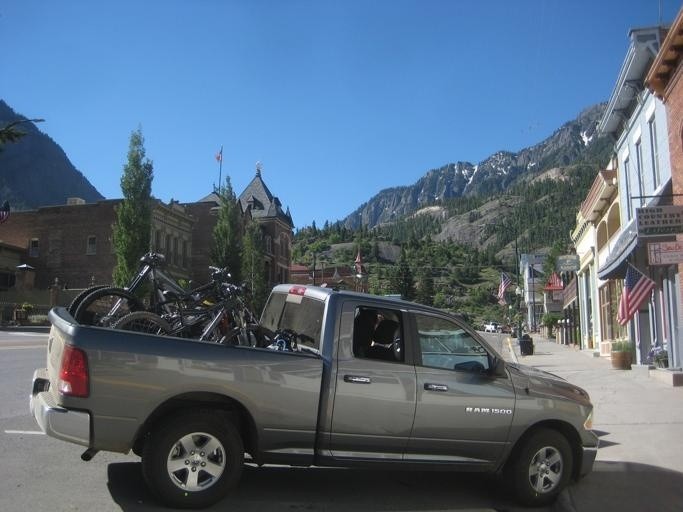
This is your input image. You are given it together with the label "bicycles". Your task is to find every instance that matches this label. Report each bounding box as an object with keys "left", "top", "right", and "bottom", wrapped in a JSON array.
[{"left": 68, "top": 252, "right": 297, "bottom": 353}]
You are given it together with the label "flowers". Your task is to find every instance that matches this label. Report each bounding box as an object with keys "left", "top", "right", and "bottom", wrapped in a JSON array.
[{"left": 647, "top": 346, "right": 668, "bottom": 359}]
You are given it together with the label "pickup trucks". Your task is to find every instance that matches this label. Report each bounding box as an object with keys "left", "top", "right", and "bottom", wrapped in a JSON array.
[{"left": 30, "top": 284, "right": 599, "bottom": 510}]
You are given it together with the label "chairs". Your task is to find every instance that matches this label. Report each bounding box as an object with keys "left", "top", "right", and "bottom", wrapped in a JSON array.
[{"left": 372, "top": 318, "right": 400, "bottom": 362}]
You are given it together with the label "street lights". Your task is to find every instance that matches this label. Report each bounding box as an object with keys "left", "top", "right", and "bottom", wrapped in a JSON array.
[{"left": 478, "top": 223, "right": 522, "bottom": 345}]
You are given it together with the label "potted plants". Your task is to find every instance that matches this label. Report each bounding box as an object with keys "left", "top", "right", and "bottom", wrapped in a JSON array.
[{"left": 610, "top": 340, "right": 633, "bottom": 371}]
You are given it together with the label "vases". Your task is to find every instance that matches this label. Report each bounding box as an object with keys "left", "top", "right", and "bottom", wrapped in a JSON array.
[{"left": 660, "top": 359, "right": 669, "bottom": 368}]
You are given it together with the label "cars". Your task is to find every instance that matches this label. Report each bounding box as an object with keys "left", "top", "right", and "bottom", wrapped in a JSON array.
[{"left": 483, "top": 321, "right": 512, "bottom": 333}]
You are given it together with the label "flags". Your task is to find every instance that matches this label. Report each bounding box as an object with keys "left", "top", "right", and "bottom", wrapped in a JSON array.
[
  {"left": 497, "top": 279, "right": 506, "bottom": 307},
  {"left": 215, "top": 147, "right": 222, "bottom": 163},
  {"left": 353, "top": 249, "right": 362, "bottom": 274},
  {"left": 616, "top": 261, "right": 656, "bottom": 327},
  {"left": 500, "top": 272, "right": 511, "bottom": 300}
]
